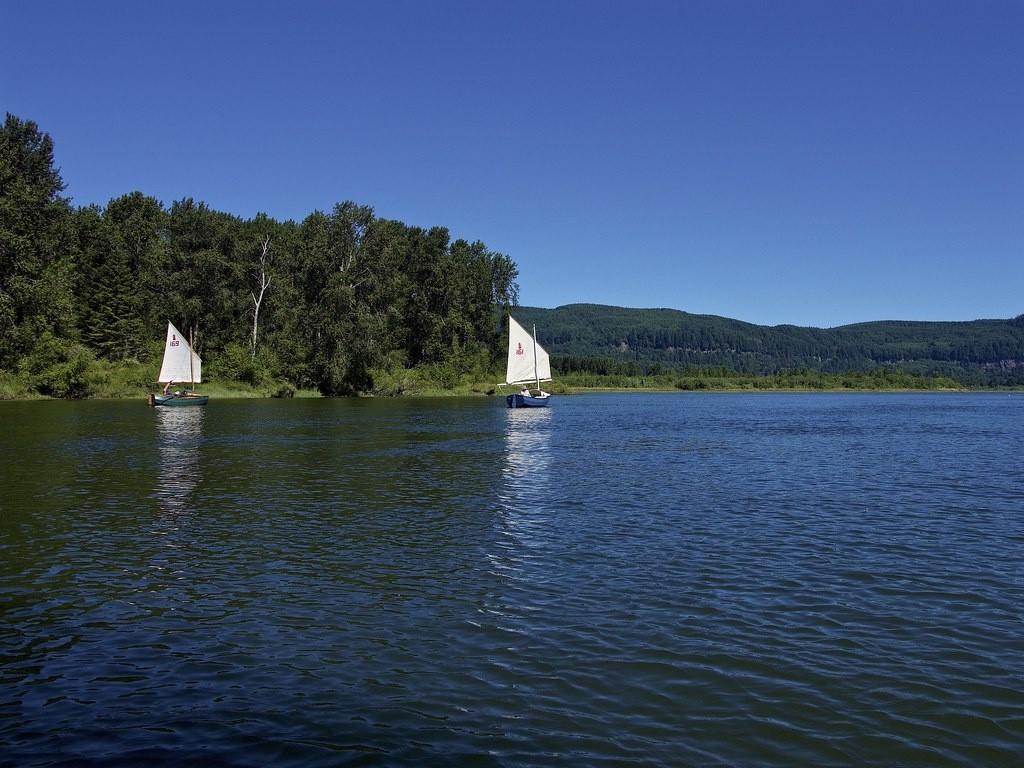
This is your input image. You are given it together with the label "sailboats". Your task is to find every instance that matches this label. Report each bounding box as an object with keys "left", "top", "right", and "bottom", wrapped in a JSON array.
[
  {"left": 147, "top": 319, "right": 210, "bottom": 406},
  {"left": 496, "top": 314, "right": 552, "bottom": 407}
]
